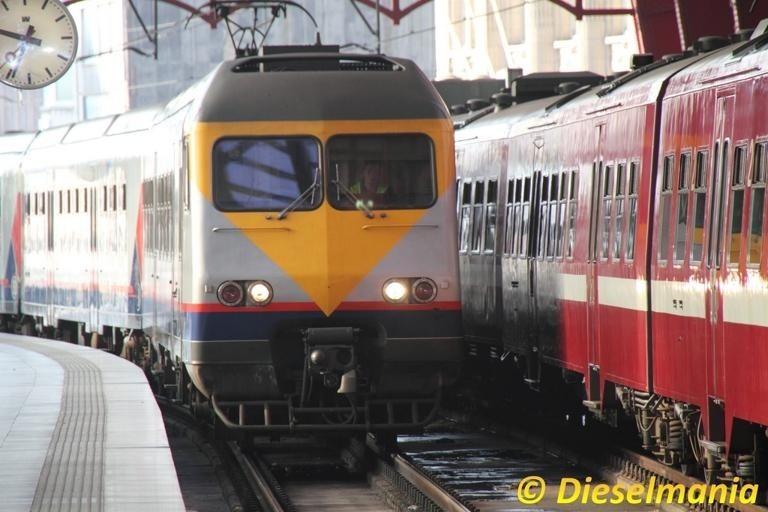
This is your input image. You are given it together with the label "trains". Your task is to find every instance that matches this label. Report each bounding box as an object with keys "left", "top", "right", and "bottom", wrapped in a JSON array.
[
  {"left": 452, "top": 32, "right": 766, "bottom": 496},
  {"left": 0, "top": 44, "right": 471, "bottom": 476}
]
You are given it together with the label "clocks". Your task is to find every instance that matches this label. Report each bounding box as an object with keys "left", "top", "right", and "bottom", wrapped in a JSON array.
[{"left": 1, "top": 0, "right": 79, "bottom": 90}]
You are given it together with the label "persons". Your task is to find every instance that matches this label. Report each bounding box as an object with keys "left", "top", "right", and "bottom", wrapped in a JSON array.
[{"left": 348, "top": 163, "right": 391, "bottom": 197}]
